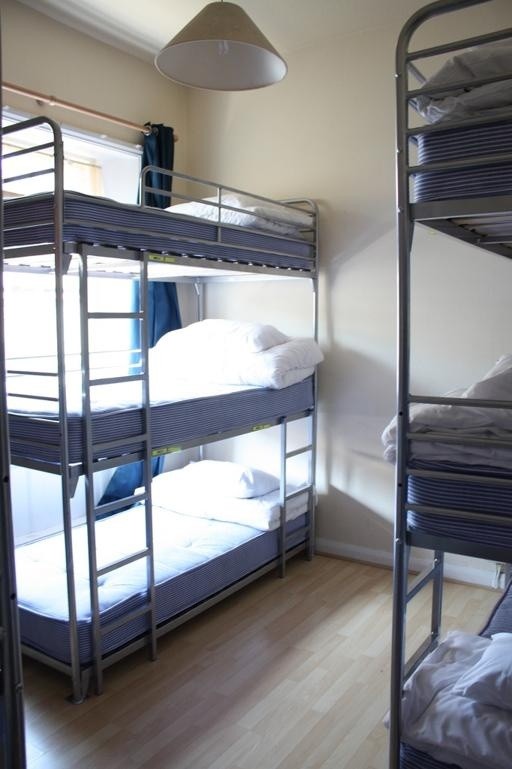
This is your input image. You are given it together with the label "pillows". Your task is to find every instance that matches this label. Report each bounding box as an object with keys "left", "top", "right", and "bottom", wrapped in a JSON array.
[
  {"left": 150, "top": 318, "right": 292, "bottom": 357},
  {"left": 173, "top": 459, "right": 287, "bottom": 501},
  {"left": 446, "top": 632, "right": 512, "bottom": 720}
]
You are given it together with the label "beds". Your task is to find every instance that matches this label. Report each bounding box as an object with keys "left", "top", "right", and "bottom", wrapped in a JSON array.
[
  {"left": 1, "top": 115, "right": 318, "bottom": 705},
  {"left": 381, "top": 1, "right": 512, "bottom": 769}
]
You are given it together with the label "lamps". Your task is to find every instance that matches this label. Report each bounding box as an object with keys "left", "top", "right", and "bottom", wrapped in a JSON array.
[{"left": 152, "top": 1, "right": 289, "bottom": 92}]
